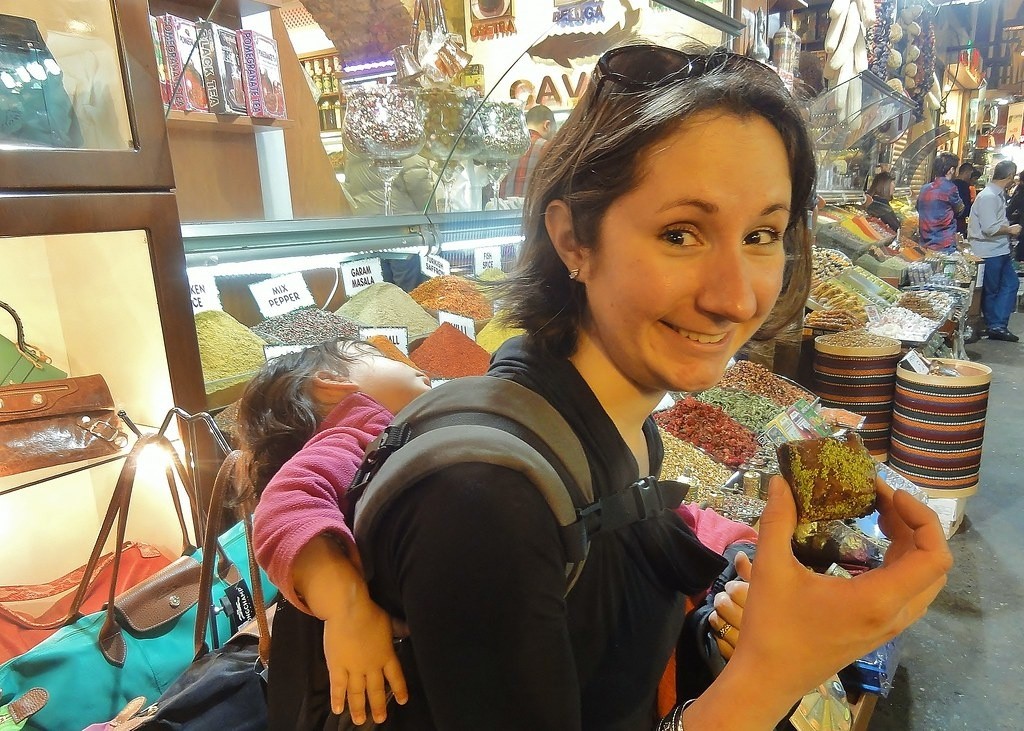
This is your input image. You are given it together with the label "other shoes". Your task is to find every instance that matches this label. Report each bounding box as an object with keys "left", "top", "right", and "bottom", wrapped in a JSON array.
[{"left": 989, "top": 326, "right": 1019, "bottom": 341}]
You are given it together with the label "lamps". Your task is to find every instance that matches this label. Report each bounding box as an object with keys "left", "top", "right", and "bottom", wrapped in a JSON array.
[{"left": 389, "top": 0, "right": 473, "bottom": 85}]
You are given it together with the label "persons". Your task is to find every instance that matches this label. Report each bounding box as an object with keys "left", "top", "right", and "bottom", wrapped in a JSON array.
[
  {"left": 487, "top": 104, "right": 556, "bottom": 199},
  {"left": 236, "top": 41, "right": 950, "bottom": 731},
  {"left": 1006, "top": 170, "right": 1024, "bottom": 276},
  {"left": 967, "top": 161, "right": 1022, "bottom": 341},
  {"left": 953, "top": 163, "right": 971, "bottom": 238},
  {"left": 969, "top": 171, "right": 982, "bottom": 205},
  {"left": 917, "top": 152, "right": 963, "bottom": 253},
  {"left": 340, "top": 145, "right": 491, "bottom": 291},
  {"left": 865, "top": 172, "right": 901, "bottom": 231}
]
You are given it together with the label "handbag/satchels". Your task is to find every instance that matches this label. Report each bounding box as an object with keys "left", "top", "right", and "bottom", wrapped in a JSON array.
[
  {"left": 0, "top": 13, "right": 83, "bottom": 148},
  {"left": 0, "top": 304, "right": 273, "bottom": 731}
]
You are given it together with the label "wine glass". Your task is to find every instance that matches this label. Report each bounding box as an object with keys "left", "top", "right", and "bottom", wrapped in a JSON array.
[{"left": 338, "top": 82, "right": 531, "bottom": 216}]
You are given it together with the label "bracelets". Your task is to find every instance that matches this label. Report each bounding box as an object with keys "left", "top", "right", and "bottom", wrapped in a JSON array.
[{"left": 656, "top": 698, "right": 696, "bottom": 731}]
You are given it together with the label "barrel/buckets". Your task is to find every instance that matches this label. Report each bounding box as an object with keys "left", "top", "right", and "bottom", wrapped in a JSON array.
[
  {"left": 813, "top": 333, "right": 902, "bottom": 463},
  {"left": 889, "top": 357, "right": 993, "bottom": 498}
]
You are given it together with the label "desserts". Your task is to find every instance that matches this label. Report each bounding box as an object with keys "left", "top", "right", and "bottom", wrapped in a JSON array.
[{"left": 776, "top": 432, "right": 878, "bottom": 525}]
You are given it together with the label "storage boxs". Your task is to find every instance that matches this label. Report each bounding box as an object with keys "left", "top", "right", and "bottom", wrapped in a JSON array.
[
  {"left": 196, "top": 21, "right": 248, "bottom": 116},
  {"left": 236, "top": 29, "right": 287, "bottom": 119},
  {"left": 150, "top": 15, "right": 171, "bottom": 110},
  {"left": 156, "top": 15, "right": 210, "bottom": 113}
]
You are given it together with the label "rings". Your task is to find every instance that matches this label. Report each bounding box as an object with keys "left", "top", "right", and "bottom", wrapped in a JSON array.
[{"left": 718, "top": 624, "right": 731, "bottom": 638}]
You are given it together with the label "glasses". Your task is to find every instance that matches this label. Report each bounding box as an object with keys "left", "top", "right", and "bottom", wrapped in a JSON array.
[{"left": 579, "top": 44, "right": 795, "bottom": 118}]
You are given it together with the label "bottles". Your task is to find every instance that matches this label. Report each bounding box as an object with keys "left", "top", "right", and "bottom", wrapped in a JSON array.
[{"left": 738, "top": 442, "right": 781, "bottom": 502}]
